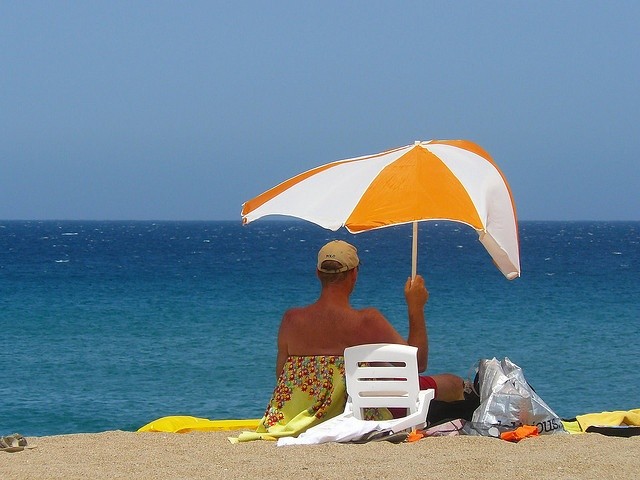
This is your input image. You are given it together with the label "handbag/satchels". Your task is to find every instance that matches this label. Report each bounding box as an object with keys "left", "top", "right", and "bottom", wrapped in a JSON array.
[{"left": 463, "top": 357, "right": 570, "bottom": 438}]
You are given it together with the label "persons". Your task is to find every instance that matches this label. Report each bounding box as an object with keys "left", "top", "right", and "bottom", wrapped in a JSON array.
[{"left": 275, "top": 241, "right": 463, "bottom": 410}]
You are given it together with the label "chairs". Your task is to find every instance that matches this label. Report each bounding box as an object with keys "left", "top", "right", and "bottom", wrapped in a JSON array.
[{"left": 306, "top": 344, "right": 435, "bottom": 444}]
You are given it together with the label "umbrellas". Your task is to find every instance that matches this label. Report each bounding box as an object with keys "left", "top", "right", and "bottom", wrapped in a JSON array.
[{"left": 241, "top": 138, "right": 521, "bottom": 298}]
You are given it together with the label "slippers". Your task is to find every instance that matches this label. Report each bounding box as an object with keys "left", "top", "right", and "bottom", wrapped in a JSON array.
[
  {"left": 14, "top": 432, "right": 37, "bottom": 449},
  {"left": 356, "top": 430, "right": 409, "bottom": 441},
  {"left": 0, "top": 436, "right": 24, "bottom": 451}
]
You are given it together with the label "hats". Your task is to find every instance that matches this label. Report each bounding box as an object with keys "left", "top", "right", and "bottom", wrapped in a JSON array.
[{"left": 317, "top": 239, "right": 359, "bottom": 273}]
